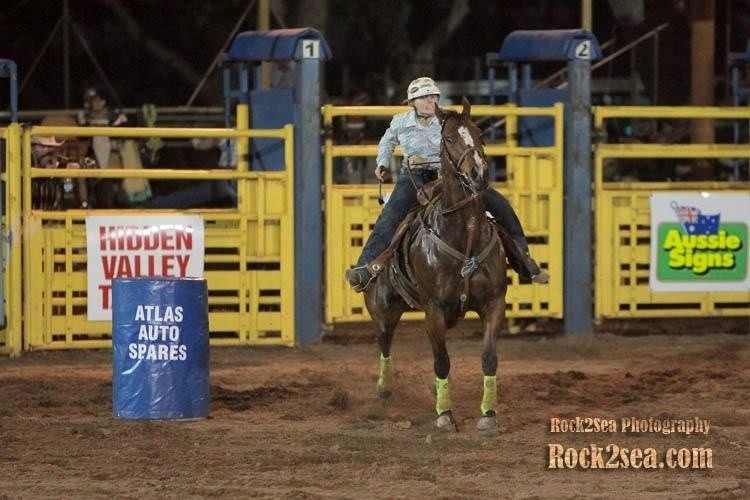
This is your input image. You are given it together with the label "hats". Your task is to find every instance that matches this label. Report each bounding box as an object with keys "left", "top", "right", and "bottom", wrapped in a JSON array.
[{"left": 407, "top": 77, "right": 440, "bottom": 100}]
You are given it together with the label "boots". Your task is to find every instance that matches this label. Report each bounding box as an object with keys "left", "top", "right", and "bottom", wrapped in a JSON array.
[
  {"left": 519, "top": 254, "right": 551, "bottom": 284},
  {"left": 346, "top": 265, "right": 372, "bottom": 293}
]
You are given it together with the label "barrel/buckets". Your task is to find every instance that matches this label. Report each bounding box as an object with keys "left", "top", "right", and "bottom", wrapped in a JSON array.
[{"left": 110, "top": 275, "right": 211, "bottom": 420}]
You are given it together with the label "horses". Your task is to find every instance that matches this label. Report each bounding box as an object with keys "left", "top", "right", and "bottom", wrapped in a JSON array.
[{"left": 362, "top": 92, "right": 511, "bottom": 438}]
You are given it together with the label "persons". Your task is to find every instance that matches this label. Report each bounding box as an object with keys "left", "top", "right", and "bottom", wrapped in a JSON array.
[
  {"left": 344, "top": 76, "right": 549, "bottom": 294},
  {"left": 337, "top": 120, "right": 394, "bottom": 184},
  {"left": 190, "top": 100, "right": 236, "bottom": 209}
]
